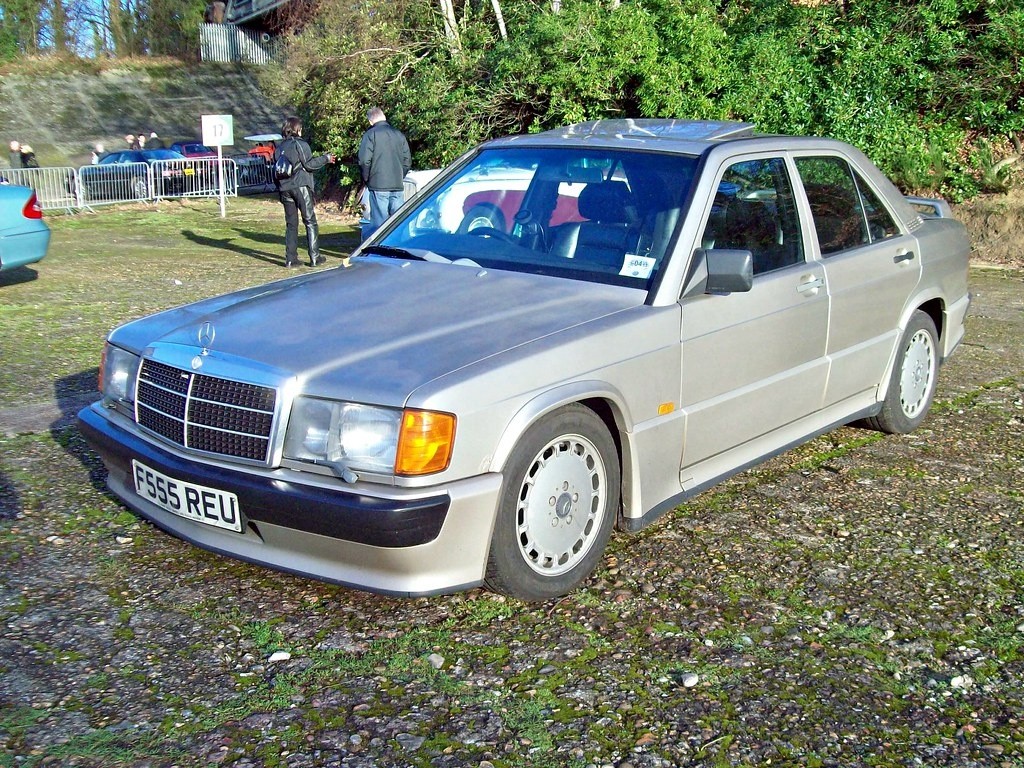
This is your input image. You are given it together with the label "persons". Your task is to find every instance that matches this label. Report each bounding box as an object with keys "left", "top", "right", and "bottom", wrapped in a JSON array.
[
  {"left": 9, "top": 140, "right": 23, "bottom": 169},
  {"left": 20, "top": 144, "right": 40, "bottom": 168},
  {"left": 145, "top": 131, "right": 167, "bottom": 151},
  {"left": 138, "top": 133, "right": 148, "bottom": 151},
  {"left": 94, "top": 143, "right": 110, "bottom": 161},
  {"left": 256, "top": 140, "right": 280, "bottom": 163},
  {"left": 358, "top": 108, "right": 412, "bottom": 244},
  {"left": 126, "top": 134, "right": 141, "bottom": 151},
  {"left": 273, "top": 117, "right": 338, "bottom": 271}
]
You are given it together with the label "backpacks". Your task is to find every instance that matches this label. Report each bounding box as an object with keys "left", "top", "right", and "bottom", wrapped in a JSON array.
[{"left": 272, "top": 139, "right": 301, "bottom": 180}]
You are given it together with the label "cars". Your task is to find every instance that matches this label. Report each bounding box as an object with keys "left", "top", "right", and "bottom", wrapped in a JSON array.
[
  {"left": 353, "top": 154, "right": 630, "bottom": 241},
  {"left": 76, "top": 120, "right": 973, "bottom": 603},
  {"left": 170, "top": 141, "right": 230, "bottom": 173},
  {"left": 0, "top": 178, "right": 52, "bottom": 274},
  {"left": 64, "top": 148, "right": 194, "bottom": 198}
]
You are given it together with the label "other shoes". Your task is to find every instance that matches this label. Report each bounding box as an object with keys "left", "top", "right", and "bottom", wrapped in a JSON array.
[
  {"left": 309, "top": 256, "right": 326, "bottom": 267},
  {"left": 285, "top": 259, "right": 303, "bottom": 268}
]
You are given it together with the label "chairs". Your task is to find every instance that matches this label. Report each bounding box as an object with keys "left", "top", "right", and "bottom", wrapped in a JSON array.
[
  {"left": 803, "top": 184, "right": 862, "bottom": 254},
  {"left": 713, "top": 199, "right": 789, "bottom": 274},
  {"left": 548, "top": 181, "right": 640, "bottom": 270}
]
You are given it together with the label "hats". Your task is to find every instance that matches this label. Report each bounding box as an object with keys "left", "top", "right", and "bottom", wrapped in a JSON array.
[
  {"left": 125, "top": 134, "right": 134, "bottom": 139},
  {"left": 151, "top": 132, "right": 156, "bottom": 138}
]
